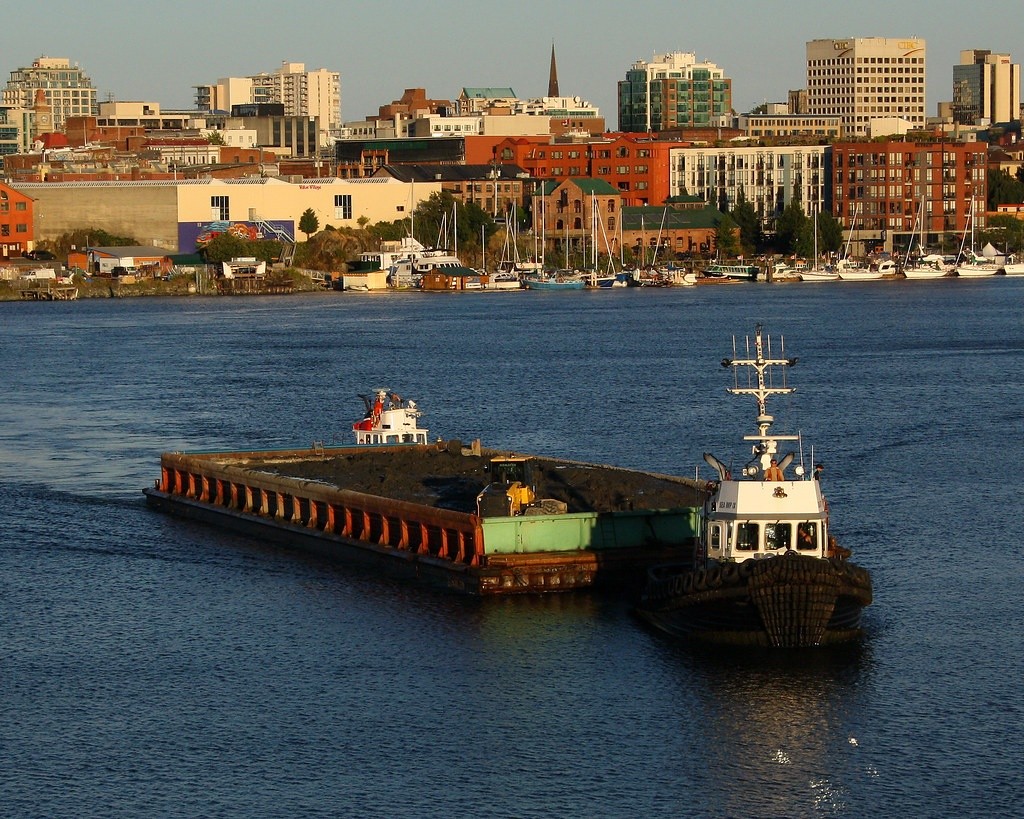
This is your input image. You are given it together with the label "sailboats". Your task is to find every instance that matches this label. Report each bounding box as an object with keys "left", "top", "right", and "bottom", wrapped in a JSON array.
[{"left": 383, "top": 179, "right": 1024, "bottom": 291}]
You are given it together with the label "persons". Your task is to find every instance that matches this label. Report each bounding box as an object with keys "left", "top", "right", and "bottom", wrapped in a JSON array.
[
  {"left": 807, "top": 464, "right": 825, "bottom": 482},
  {"left": 764, "top": 458, "right": 785, "bottom": 482}
]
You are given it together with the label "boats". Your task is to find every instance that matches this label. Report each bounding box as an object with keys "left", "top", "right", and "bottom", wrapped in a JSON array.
[{"left": 138, "top": 322, "right": 873, "bottom": 651}]
